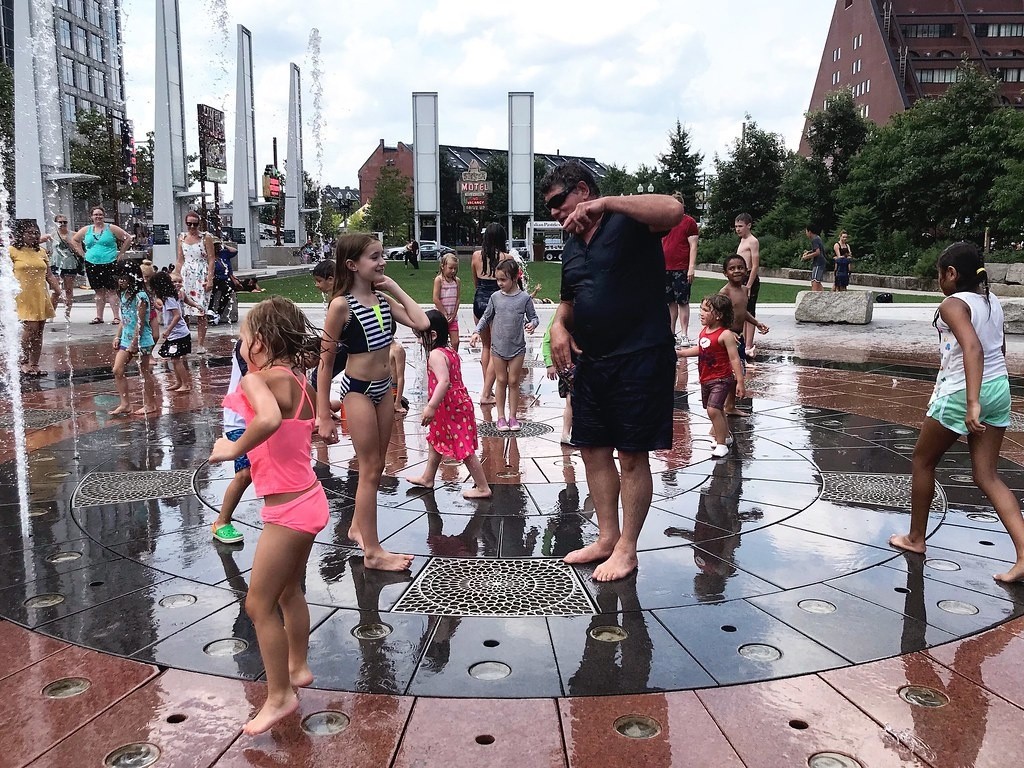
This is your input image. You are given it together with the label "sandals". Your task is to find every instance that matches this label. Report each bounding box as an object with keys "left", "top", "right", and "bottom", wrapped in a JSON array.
[
  {"left": 20, "top": 360, "right": 37, "bottom": 374},
  {"left": 212, "top": 521, "right": 243, "bottom": 543},
  {"left": 30, "top": 364, "right": 48, "bottom": 376}
]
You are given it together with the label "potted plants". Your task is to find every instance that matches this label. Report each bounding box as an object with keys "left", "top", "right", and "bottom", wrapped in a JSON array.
[{"left": 533, "top": 233, "right": 546, "bottom": 261}]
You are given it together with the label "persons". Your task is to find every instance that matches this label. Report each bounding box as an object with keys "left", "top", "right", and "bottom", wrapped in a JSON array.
[
  {"left": 890, "top": 243, "right": 1024, "bottom": 582},
  {"left": 301, "top": 235, "right": 332, "bottom": 263},
  {"left": 735, "top": 213, "right": 760, "bottom": 353},
  {"left": 719, "top": 254, "right": 770, "bottom": 417},
  {"left": 294, "top": 260, "right": 348, "bottom": 434},
  {"left": 433, "top": 253, "right": 460, "bottom": 350},
  {"left": 212, "top": 339, "right": 252, "bottom": 544},
  {"left": 831, "top": 229, "right": 855, "bottom": 292},
  {"left": 675, "top": 295, "right": 746, "bottom": 456},
  {"left": 538, "top": 162, "right": 685, "bottom": 582},
  {"left": 315, "top": 235, "right": 430, "bottom": 574},
  {"left": 471, "top": 221, "right": 543, "bottom": 430},
  {"left": 228, "top": 277, "right": 261, "bottom": 292},
  {"left": 405, "top": 308, "right": 492, "bottom": 498},
  {"left": 405, "top": 236, "right": 419, "bottom": 269},
  {"left": 663, "top": 191, "right": 700, "bottom": 348},
  {"left": 209, "top": 296, "right": 330, "bottom": 736},
  {"left": 802, "top": 225, "right": 823, "bottom": 290},
  {"left": 543, "top": 311, "right": 575, "bottom": 447},
  {"left": 389, "top": 323, "right": 407, "bottom": 413},
  {"left": 0, "top": 207, "right": 239, "bottom": 414}
]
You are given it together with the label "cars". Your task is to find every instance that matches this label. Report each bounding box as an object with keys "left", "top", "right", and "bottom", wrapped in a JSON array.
[{"left": 386, "top": 240, "right": 458, "bottom": 260}]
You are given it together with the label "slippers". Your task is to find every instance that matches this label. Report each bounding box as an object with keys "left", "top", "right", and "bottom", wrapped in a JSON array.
[
  {"left": 90, "top": 318, "right": 104, "bottom": 324},
  {"left": 111, "top": 318, "right": 120, "bottom": 325}
]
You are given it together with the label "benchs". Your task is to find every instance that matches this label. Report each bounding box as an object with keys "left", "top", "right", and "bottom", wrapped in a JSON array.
[{"left": 189, "top": 291, "right": 238, "bottom": 324}]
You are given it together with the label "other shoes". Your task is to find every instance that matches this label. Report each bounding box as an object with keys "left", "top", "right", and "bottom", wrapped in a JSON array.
[
  {"left": 711, "top": 436, "right": 733, "bottom": 447},
  {"left": 509, "top": 417, "right": 521, "bottom": 431},
  {"left": 712, "top": 444, "right": 729, "bottom": 457},
  {"left": 561, "top": 437, "right": 575, "bottom": 445},
  {"left": 680, "top": 335, "right": 690, "bottom": 347},
  {"left": 196, "top": 346, "right": 205, "bottom": 353},
  {"left": 206, "top": 309, "right": 214, "bottom": 316},
  {"left": 214, "top": 314, "right": 221, "bottom": 325},
  {"left": 496, "top": 418, "right": 509, "bottom": 431}
]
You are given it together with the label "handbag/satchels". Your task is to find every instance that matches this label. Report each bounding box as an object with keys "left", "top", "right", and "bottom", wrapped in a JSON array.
[
  {"left": 231, "top": 275, "right": 243, "bottom": 289},
  {"left": 77, "top": 262, "right": 84, "bottom": 276}
]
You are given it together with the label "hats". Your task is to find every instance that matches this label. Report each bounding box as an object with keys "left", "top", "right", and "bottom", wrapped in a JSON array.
[{"left": 211, "top": 236, "right": 221, "bottom": 245}]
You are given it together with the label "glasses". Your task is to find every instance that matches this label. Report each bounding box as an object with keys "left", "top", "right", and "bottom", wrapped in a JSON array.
[
  {"left": 187, "top": 222, "right": 199, "bottom": 226},
  {"left": 59, "top": 221, "right": 67, "bottom": 224},
  {"left": 544, "top": 184, "right": 576, "bottom": 212},
  {"left": 23, "top": 231, "right": 40, "bottom": 234}
]
